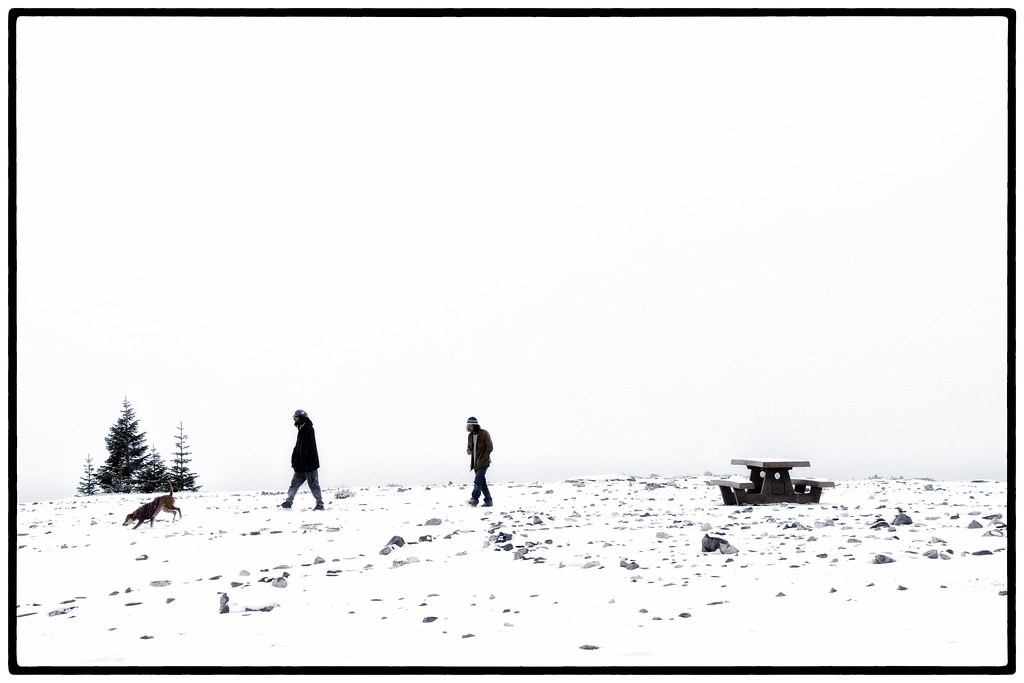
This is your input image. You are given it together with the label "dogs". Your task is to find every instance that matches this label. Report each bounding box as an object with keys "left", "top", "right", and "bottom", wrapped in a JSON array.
[{"left": 122, "top": 480, "right": 182, "bottom": 530}]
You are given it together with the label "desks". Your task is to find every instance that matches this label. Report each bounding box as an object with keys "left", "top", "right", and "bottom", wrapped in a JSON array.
[{"left": 719, "top": 458, "right": 822, "bottom": 505}]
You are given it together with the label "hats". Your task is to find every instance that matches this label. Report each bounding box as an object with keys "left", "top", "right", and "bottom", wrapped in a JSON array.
[
  {"left": 467, "top": 417, "right": 479, "bottom": 425},
  {"left": 292, "top": 410, "right": 307, "bottom": 416}
]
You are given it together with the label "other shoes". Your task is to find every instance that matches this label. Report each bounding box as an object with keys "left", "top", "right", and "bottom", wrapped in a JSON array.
[
  {"left": 277, "top": 503, "right": 291, "bottom": 508},
  {"left": 314, "top": 505, "right": 324, "bottom": 510},
  {"left": 482, "top": 500, "right": 493, "bottom": 506},
  {"left": 469, "top": 500, "right": 477, "bottom": 507}
]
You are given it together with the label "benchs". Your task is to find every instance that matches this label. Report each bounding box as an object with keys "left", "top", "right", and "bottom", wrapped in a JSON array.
[
  {"left": 710, "top": 478, "right": 755, "bottom": 490},
  {"left": 791, "top": 478, "right": 835, "bottom": 487}
]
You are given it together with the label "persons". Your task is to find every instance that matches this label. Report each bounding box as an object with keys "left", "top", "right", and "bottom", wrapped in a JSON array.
[
  {"left": 276, "top": 410, "right": 324, "bottom": 511},
  {"left": 466, "top": 417, "right": 493, "bottom": 507}
]
